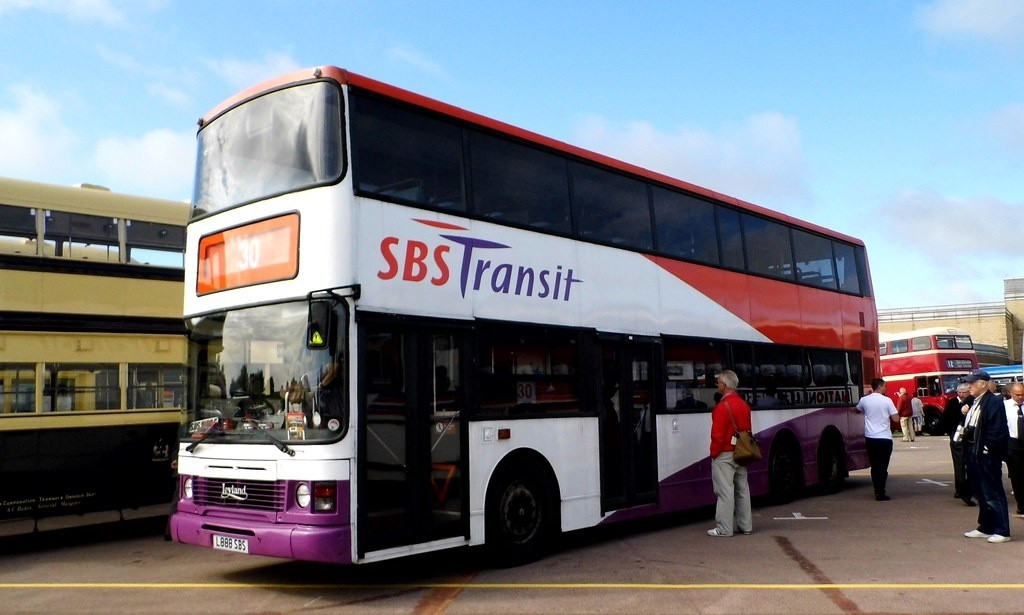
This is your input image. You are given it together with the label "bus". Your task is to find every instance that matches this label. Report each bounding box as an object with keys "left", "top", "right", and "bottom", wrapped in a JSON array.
[
  {"left": 879, "top": 327, "right": 979, "bottom": 436},
  {"left": 166, "top": 65, "right": 883, "bottom": 569},
  {"left": 0, "top": 177, "right": 285, "bottom": 537}
]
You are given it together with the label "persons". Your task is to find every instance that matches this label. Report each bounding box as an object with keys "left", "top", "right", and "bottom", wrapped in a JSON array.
[
  {"left": 961, "top": 370, "right": 1011, "bottom": 543},
  {"left": 894, "top": 388, "right": 915, "bottom": 442},
  {"left": 852, "top": 378, "right": 900, "bottom": 501},
  {"left": 910, "top": 392, "right": 925, "bottom": 436},
  {"left": 1002, "top": 382, "right": 1024, "bottom": 518},
  {"left": 305, "top": 351, "right": 344, "bottom": 430},
  {"left": 706, "top": 369, "right": 753, "bottom": 537},
  {"left": 997, "top": 382, "right": 1012, "bottom": 401},
  {"left": 988, "top": 381, "right": 997, "bottom": 393},
  {"left": 940, "top": 382, "right": 974, "bottom": 499}
]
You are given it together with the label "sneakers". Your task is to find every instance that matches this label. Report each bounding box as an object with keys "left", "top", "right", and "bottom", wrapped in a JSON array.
[
  {"left": 744, "top": 530, "right": 752, "bottom": 535},
  {"left": 707, "top": 528, "right": 733, "bottom": 537}
]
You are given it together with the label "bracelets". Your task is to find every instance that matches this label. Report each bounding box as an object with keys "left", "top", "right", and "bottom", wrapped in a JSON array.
[{"left": 318, "top": 382, "right": 327, "bottom": 389}]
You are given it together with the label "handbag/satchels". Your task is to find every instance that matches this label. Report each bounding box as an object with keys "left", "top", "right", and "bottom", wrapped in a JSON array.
[{"left": 733, "top": 431, "right": 762, "bottom": 467}]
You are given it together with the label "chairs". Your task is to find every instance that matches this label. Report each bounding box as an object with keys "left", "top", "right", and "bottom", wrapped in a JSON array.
[
  {"left": 371, "top": 176, "right": 841, "bottom": 292},
  {"left": 695, "top": 361, "right": 849, "bottom": 388}
]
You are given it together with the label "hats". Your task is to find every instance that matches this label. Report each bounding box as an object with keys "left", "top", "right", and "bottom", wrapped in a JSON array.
[{"left": 963, "top": 370, "right": 990, "bottom": 381}]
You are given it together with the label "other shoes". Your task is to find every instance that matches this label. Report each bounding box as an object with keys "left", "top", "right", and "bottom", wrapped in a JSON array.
[
  {"left": 986, "top": 534, "right": 1011, "bottom": 543},
  {"left": 876, "top": 492, "right": 890, "bottom": 501},
  {"left": 960, "top": 493, "right": 976, "bottom": 506},
  {"left": 955, "top": 492, "right": 961, "bottom": 498},
  {"left": 964, "top": 530, "right": 991, "bottom": 538}
]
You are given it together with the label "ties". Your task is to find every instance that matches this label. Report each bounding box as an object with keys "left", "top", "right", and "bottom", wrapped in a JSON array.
[{"left": 1016, "top": 403, "right": 1024, "bottom": 440}]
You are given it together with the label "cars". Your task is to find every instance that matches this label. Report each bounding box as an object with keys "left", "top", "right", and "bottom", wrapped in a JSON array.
[{"left": 979, "top": 365, "right": 1023, "bottom": 384}]
